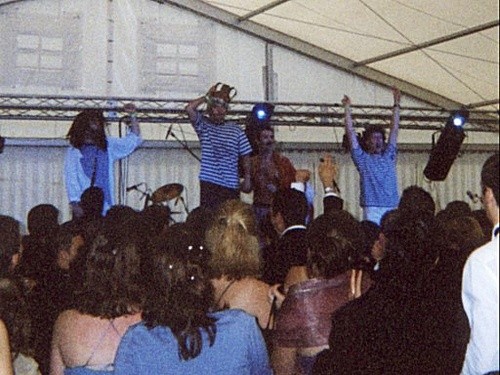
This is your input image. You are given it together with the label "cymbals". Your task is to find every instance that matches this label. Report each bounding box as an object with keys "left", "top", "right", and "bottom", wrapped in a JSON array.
[{"left": 151, "top": 182, "right": 185, "bottom": 204}]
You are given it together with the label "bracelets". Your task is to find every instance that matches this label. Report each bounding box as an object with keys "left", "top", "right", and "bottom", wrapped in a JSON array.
[
  {"left": 243, "top": 175, "right": 251, "bottom": 178},
  {"left": 130, "top": 111, "right": 137, "bottom": 117},
  {"left": 393, "top": 104, "right": 400, "bottom": 107},
  {"left": 201, "top": 96, "right": 208, "bottom": 102}
]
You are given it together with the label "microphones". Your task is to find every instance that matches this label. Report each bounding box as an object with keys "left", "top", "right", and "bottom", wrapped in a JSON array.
[
  {"left": 166, "top": 124, "right": 172, "bottom": 138},
  {"left": 467, "top": 190, "right": 478, "bottom": 203},
  {"left": 127, "top": 184, "right": 141, "bottom": 191}
]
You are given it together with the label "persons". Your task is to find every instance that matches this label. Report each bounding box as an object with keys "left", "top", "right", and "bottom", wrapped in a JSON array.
[
  {"left": 239, "top": 127, "right": 291, "bottom": 218},
  {"left": 0, "top": 147, "right": 500, "bottom": 375},
  {"left": 64, "top": 103, "right": 142, "bottom": 217},
  {"left": 185, "top": 86, "right": 251, "bottom": 209},
  {"left": 342, "top": 84, "right": 401, "bottom": 225}
]
